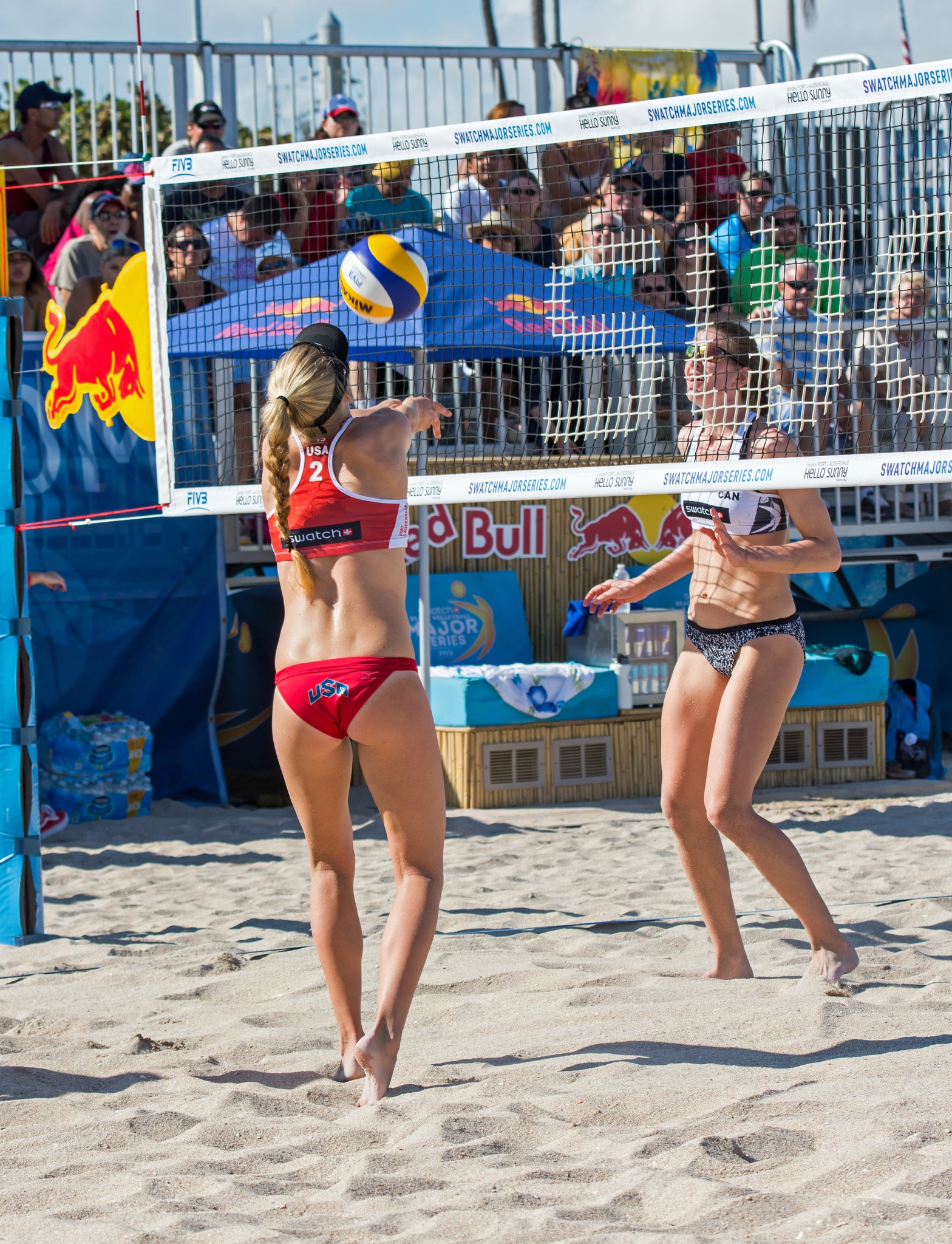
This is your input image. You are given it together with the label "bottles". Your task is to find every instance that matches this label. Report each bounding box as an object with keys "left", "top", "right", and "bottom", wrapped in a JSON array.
[
  {"left": 613, "top": 562, "right": 631, "bottom": 615},
  {"left": 37, "top": 711, "right": 154, "bottom": 824}
]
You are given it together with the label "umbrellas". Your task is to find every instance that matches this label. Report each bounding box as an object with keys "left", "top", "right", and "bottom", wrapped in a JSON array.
[{"left": 170, "top": 226, "right": 697, "bottom": 706}]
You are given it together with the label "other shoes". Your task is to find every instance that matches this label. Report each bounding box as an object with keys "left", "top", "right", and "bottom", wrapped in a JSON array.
[{"left": 888, "top": 500, "right": 952, "bottom": 519}]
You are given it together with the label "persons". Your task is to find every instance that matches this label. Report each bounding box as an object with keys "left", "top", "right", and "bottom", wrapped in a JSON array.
[
  {"left": 2, "top": 82, "right": 951, "bottom": 451},
  {"left": 581, "top": 318, "right": 858, "bottom": 983},
  {"left": 259, "top": 325, "right": 452, "bottom": 1104}
]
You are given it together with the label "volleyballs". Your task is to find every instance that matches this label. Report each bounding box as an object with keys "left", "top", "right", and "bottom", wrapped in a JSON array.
[{"left": 337, "top": 233, "right": 430, "bottom": 326}]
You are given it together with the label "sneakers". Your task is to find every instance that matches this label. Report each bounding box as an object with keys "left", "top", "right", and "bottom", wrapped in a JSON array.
[{"left": 860, "top": 489, "right": 890, "bottom": 520}]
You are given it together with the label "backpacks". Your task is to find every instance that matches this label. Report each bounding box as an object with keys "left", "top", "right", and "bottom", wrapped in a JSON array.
[{"left": 884, "top": 677, "right": 948, "bottom": 782}]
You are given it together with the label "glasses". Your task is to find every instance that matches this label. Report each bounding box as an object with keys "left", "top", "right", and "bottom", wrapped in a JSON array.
[
  {"left": 641, "top": 285, "right": 666, "bottom": 293},
  {"left": 509, "top": 187, "right": 537, "bottom": 197},
  {"left": 766, "top": 216, "right": 800, "bottom": 226},
  {"left": 97, "top": 209, "right": 127, "bottom": 222},
  {"left": 484, "top": 231, "right": 514, "bottom": 242},
  {"left": 593, "top": 224, "right": 621, "bottom": 233},
  {"left": 786, "top": 280, "right": 817, "bottom": 292},
  {"left": 40, "top": 100, "right": 63, "bottom": 110},
  {"left": 611, "top": 186, "right": 641, "bottom": 197},
  {"left": 333, "top": 113, "right": 356, "bottom": 125},
  {"left": 744, "top": 190, "right": 773, "bottom": 200},
  {"left": 258, "top": 259, "right": 288, "bottom": 276},
  {"left": 198, "top": 121, "right": 223, "bottom": 131},
  {"left": 677, "top": 236, "right": 706, "bottom": 248},
  {"left": 111, "top": 239, "right": 140, "bottom": 253},
  {"left": 172, "top": 239, "right": 207, "bottom": 250},
  {"left": 685, "top": 344, "right": 748, "bottom": 369}
]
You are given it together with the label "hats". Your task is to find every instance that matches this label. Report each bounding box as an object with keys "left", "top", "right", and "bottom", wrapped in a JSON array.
[
  {"left": 465, "top": 210, "right": 534, "bottom": 248},
  {"left": 372, "top": 159, "right": 416, "bottom": 181},
  {"left": 285, "top": 322, "right": 348, "bottom": 431},
  {"left": 187, "top": 101, "right": 224, "bottom": 122},
  {"left": 762, "top": 195, "right": 800, "bottom": 217},
  {"left": 16, "top": 81, "right": 72, "bottom": 109},
  {"left": 90, "top": 194, "right": 128, "bottom": 219},
  {"left": 254, "top": 241, "right": 293, "bottom": 267},
  {"left": 324, "top": 93, "right": 359, "bottom": 118},
  {"left": 608, "top": 167, "right": 643, "bottom": 186},
  {"left": 7, "top": 235, "right": 36, "bottom": 261}
]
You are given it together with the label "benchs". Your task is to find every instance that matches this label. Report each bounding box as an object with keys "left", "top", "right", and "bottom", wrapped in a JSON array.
[
  {"left": 405, "top": 571, "right": 619, "bottom": 809},
  {"left": 626, "top": 567, "right": 889, "bottom": 785}
]
